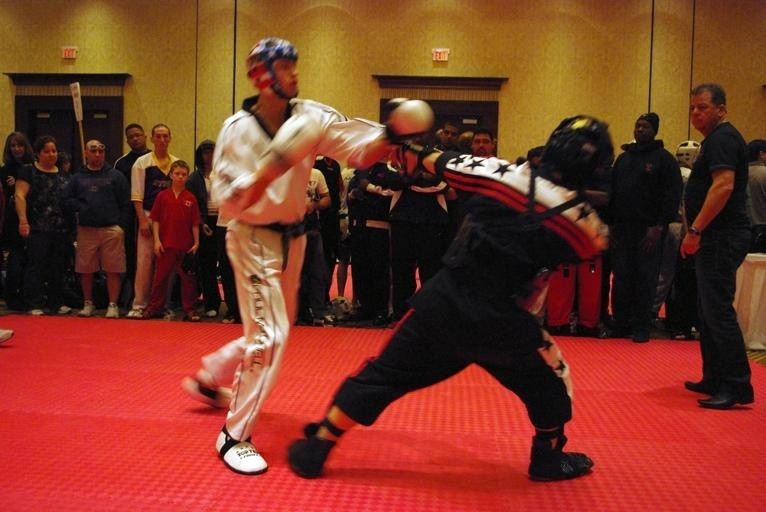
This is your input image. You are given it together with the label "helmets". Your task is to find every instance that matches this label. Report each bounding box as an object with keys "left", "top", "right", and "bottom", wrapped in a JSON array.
[
  {"left": 538, "top": 115, "right": 615, "bottom": 193},
  {"left": 247, "top": 37, "right": 296, "bottom": 71},
  {"left": 677, "top": 140, "right": 701, "bottom": 169}
]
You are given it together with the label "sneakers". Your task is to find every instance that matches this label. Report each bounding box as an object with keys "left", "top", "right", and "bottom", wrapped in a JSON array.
[
  {"left": 127, "top": 305, "right": 147, "bottom": 319},
  {"left": 183, "top": 368, "right": 232, "bottom": 409},
  {"left": 77, "top": 300, "right": 96, "bottom": 317},
  {"left": 206, "top": 309, "right": 217, "bottom": 317},
  {"left": 32, "top": 309, "right": 43, "bottom": 315},
  {"left": 106, "top": 303, "right": 120, "bottom": 319},
  {"left": 187, "top": 312, "right": 201, "bottom": 321},
  {"left": 529, "top": 434, "right": 594, "bottom": 480},
  {"left": 222, "top": 315, "right": 237, "bottom": 324},
  {"left": 287, "top": 424, "right": 335, "bottom": 478},
  {"left": 1, "top": 329, "right": 13, "bottom": 343},
  {"left": 216, "top": 431, "right": 268, "bottom": 475},
  {"left": 58, "top": 306, "right": 70, "bottom": 313}
]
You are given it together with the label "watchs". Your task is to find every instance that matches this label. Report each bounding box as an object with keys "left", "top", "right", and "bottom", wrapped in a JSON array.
[{"left": 687, "top": 227, "right": 702, "bottom": 238}]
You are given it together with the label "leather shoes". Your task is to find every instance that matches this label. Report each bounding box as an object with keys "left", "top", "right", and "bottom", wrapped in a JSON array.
[
  {"left": 322, "top": 315, "right": 336, "bottom": 324},
  {"left": 698, "top": 392, "right": 753, "bottom": 407},
  {"left": 633, "top": 328, "right": 650, "bottom": 343},
  {"left": 686, "top": 380, "right": 718, "bottom": 394}
]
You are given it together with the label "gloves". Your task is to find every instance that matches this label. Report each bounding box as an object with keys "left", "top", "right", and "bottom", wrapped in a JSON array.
[
  {"left": 255, "top": 113, "right": 322, "bottom": 182},
  {"left": 387, "top": 102, "right": 434, "bottom": 142}
]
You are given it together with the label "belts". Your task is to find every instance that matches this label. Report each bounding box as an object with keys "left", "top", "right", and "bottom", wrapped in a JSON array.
[{"left": 261, "top": 222, "right": 306, "bottom": 270}]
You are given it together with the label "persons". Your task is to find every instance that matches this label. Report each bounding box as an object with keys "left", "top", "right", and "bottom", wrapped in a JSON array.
[
  {"left": 680, "top": 82, "right": 754, "bottom": 408},
  {"left": 388, "top": 181, "right": 457, "bottom": 319},
  {"left": 287, "top": 95, "right": 615, "bottom": 484},
  {"left": 604, "top": 113, "right": 684, "bottom": 345},
  {"left": 313, "top": 156, "right": 344, "bottom": 314},
  {"left": 296, "top": 167, "right": 331, "bottom": 325},
  {"left": 142, "top": 161, "right": 201, "bottom": 321},
  {"left": 55, "top": 151, "right": 72, "bottom": 174},
  {"left": 439, "top": 114, "right": 463, "bottom": 152},
  {"left": 181, "top": 39, "right": 435, "bottom": 473},
  {"left": 0, "top": 133, "right": 32, "bottom": 312},
  {"left": 114, "top": 124, "right": 152, "bottom": 180},
  {"left": 14, "top": 137, "right": 72, "bottom": 316},
  {"left": 334, "top": 165, "right": 356, "bottom": 303},
  {"left": 747, "top": 139, "right": 766, "bottom": 252},
  {"left": 669, "top": 142, "right": 703, "bottom": 339},
  {"left": 472, "top": 129, "right": 497, "bottom": 157},
  {"left": 546, "top": 257, "right": 601, "bottom": 337},
  {"left": 62, "top": 140, "right": 132, "bottom": 317},
  {"left": 186, "top": 140, "right": 238, "bottom": 323},
  {"left": 126, "top": 124, "right": 178, "bottom": 319}
]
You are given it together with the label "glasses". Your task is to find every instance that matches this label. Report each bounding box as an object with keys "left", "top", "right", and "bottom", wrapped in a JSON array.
[{"left": 91, "top": 144, "right": 105, "bottom": 149}]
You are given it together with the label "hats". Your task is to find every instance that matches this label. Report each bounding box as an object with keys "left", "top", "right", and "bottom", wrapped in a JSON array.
[{"left": 638, "top": 112, "right": 659, "bottom": 131}]
